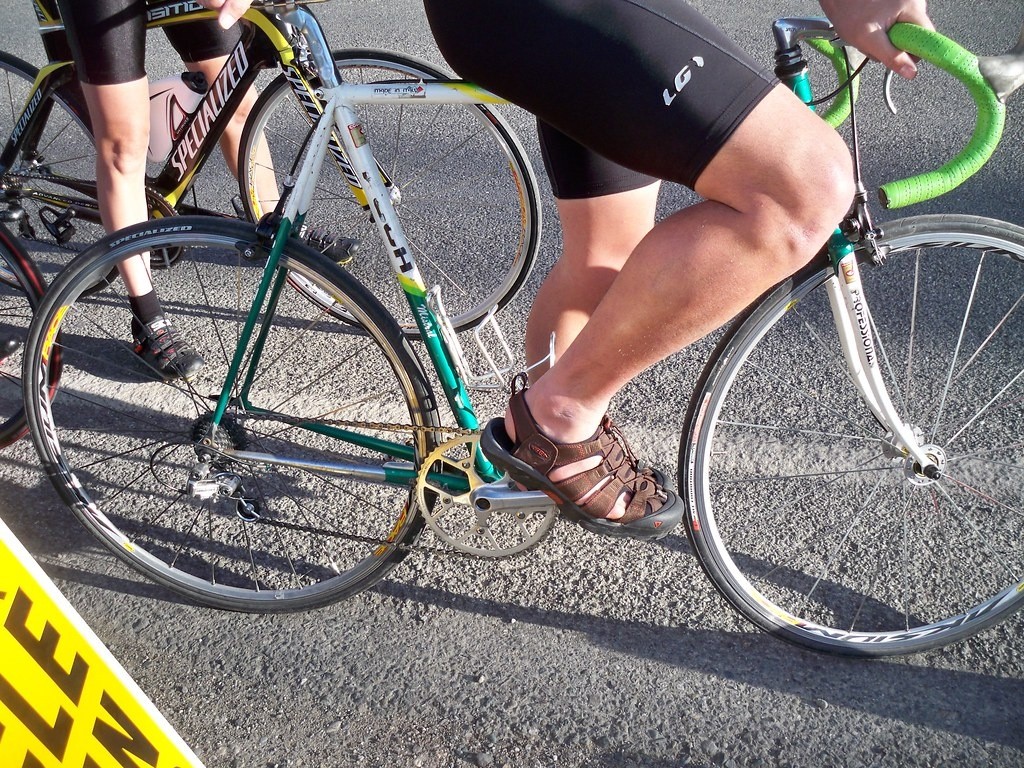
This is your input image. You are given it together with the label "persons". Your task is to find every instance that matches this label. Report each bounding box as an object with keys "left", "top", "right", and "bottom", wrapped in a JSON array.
[
  {"left": 424, "top": 0, "right": 936, "bottom": 540},
  {"left": 61, "top": 1, "right": 362, "bottom": 379}
]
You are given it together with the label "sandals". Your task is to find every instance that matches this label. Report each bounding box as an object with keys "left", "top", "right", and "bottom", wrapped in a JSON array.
[{"left": 480, "top": 372, "right": 684, "bottom": 540}]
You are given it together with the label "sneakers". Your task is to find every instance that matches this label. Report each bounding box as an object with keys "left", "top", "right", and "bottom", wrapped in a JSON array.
[
  {"left": 299, "top": 226, "right": 362, "bottom": 266},
  {"left": 130, "top": 313, "right": 206, "bottom": 381}
]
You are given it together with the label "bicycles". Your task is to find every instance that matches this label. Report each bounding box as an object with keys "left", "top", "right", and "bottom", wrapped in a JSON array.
[
  {"left": 24, "top": 0, "right": 1024, "bottom": 657},
  {"left": 0, "top": 0, "right": 548, "bottom": 454}
]
